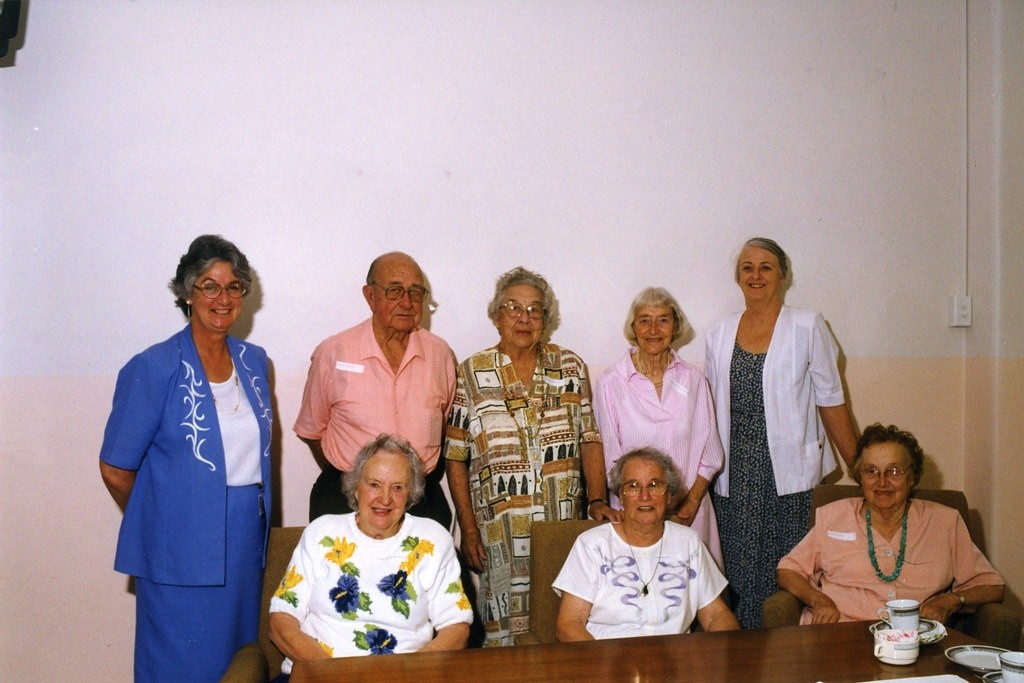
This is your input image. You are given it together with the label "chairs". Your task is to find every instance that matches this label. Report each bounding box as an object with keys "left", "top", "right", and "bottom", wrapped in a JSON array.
[
  {"left": 763, "top": 484, "right": 1021, "bottom": 654},
  {"left": 218, "top": 526, "right": 308, "bottom": 683},
  {"left": 514, "top": 514, "right": 703, "bottom": 647}
]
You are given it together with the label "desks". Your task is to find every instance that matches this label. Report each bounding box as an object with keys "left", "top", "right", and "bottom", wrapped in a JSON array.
[{"left": 289, "top": 615, "right": 998, "bottom": 683}]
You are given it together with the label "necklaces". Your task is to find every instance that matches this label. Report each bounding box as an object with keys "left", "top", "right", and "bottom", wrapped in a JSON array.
[
  {"left": 212, "top": 368, "right": 241, "bottom": 414},
  {"left": 623, "top": 522, "right": 663, "bottom": 596},
  {"left": 865, "top": 507, "right": 907, "bottom": 584},
  {"left": 498, "top": 342, "right": 544, "bottom": 439}
]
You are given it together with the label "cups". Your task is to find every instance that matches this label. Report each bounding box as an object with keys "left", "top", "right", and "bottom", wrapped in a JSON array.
[
  {"left": 873, "top": 629, "right": 920, "bottom": 665},
  {"left": 999, "top": 651, "right": 1024, "bottom": 683},
  {"left": 878, "top": 599, "right": 920, "bottom": 631}
]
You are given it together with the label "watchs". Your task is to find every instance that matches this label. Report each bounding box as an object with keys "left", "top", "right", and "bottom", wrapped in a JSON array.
[{"left": 953, "top": 592, "right": 965, "bottom": 608}]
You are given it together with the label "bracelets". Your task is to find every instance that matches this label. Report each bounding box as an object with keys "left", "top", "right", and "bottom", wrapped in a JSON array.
[{"left": 590, "top": 498, "right": 608, "bottom": 506}]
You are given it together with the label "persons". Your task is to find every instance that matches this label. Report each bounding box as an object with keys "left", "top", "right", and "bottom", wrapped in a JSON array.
[
  {"left": 777, "top": 422, "right": 1006, "bottom": 626},
  {"left": 593, "top": 287, "right": 725, "bottom": 575},
  {"left": 269, "top": 433, "right": 474, "bottom": 683},
  {"left": 445, "top": 267, "right": 624, "bottom": 648},
  {"left": 551, "top": 446, "right": 741, "bottom": 642},
  {"left": 292, "top": 251, "right": 457, "bottom": 532},
  {"left": 99, "top": 235, "right": 274, "bottom": 683},
  {"left": 703, "top": 237, "right": 858, "bottom": 631}
]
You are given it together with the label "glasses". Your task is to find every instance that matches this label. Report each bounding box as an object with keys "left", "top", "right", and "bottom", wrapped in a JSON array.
[
  {"left": 373, "top": 281, "right": 429, "bottom": 303},
  {"left": 193, "top": 282, "right": 247, "bottom": 300},
  {"left": 858, "top": 465, "right": 914, "bottom": 478},
  {"left": 618, "top": 479, "right": 673, "bottom": 497},
  {"left": 497, "top": 300, "right": 547, "bottom": 321}
]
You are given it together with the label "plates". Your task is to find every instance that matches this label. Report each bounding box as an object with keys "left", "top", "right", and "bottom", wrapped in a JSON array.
[
  {"left": 869, "top": 618, "right": 947, "bottom": 646},
  {"left": 982, "top": 670, "right": 1003, "bottom": 683},
  {"left": 944, "top": 644, "right": 1011, "bottom": 673}
]
